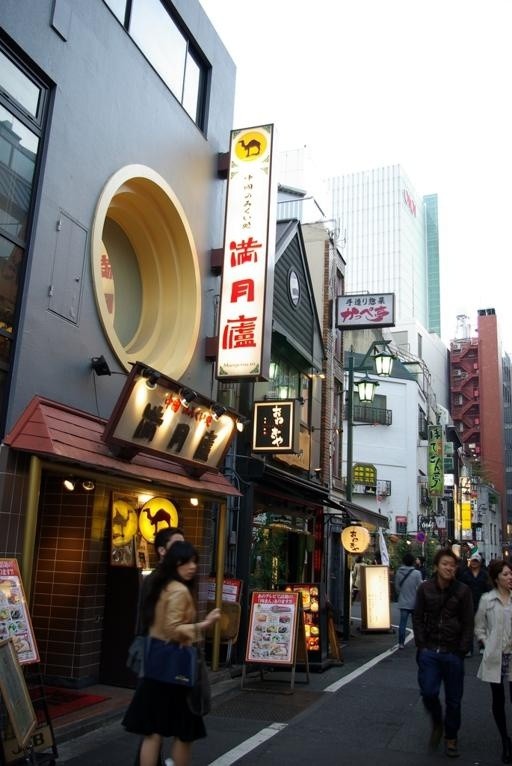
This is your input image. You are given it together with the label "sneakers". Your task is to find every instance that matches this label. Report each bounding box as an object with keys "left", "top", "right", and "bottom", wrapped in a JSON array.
[
  {"left": 446, "top": 739, "right": 459, "bottom": 756},
  {"left": 429, "top": 723, "right": 443, "bottom": 746}
]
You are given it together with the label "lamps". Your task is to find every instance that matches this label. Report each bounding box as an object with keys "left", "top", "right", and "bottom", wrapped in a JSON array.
[{"left": 91, "top": 354, "right": 251, "bottom": 432}]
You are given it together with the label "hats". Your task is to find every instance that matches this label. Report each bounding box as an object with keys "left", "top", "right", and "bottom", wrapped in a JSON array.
[{"left": 471, "top": 554, "right": 481, "bottom": 562}]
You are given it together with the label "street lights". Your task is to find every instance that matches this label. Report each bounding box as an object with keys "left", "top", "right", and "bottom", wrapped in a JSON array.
[
  {"left": 343, "top": 340, "right": 397, "bottom": 640},
  {"left": 457, "top": 478, "right": 472, "bottom": 557}
]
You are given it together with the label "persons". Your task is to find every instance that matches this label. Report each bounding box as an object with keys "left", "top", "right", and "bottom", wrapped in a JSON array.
[
  {"left": 472, "top": 559, "right": 512, "bottom": 761},
  {"left": 393, "top": 554, "right": 426, "bottom": 649},
  {"left": 412, "top": 549, "right": 476, "bottom": 756},
  {"left": 455, "top": 551, "right": 492, "bottom": 657},
  {"left": 120, "top": 540, "right": 222, "bottom": 766},
  {"left": 351, "top": 556, "right": 368, "bottom": 606},
  {"left": 130, "top": 527, "right": 185, "bottom": 766},
  {"left": 415, "top": 557, "right": 427, "bottom": 579}
]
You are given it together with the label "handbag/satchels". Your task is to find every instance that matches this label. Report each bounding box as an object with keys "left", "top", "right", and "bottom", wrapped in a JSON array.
[{"left": 143, "top": 636, "right": 199, "bottom": 687}]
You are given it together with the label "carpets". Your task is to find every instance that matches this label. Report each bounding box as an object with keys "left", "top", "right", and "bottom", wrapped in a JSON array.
[{"left": 28, "top": 687, "right": 112, "bottom": 724}]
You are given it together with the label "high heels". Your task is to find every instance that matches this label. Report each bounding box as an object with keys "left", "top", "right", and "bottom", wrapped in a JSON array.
[{"left": 502, "top": 737, "right": 512, "bottom": 764}]
